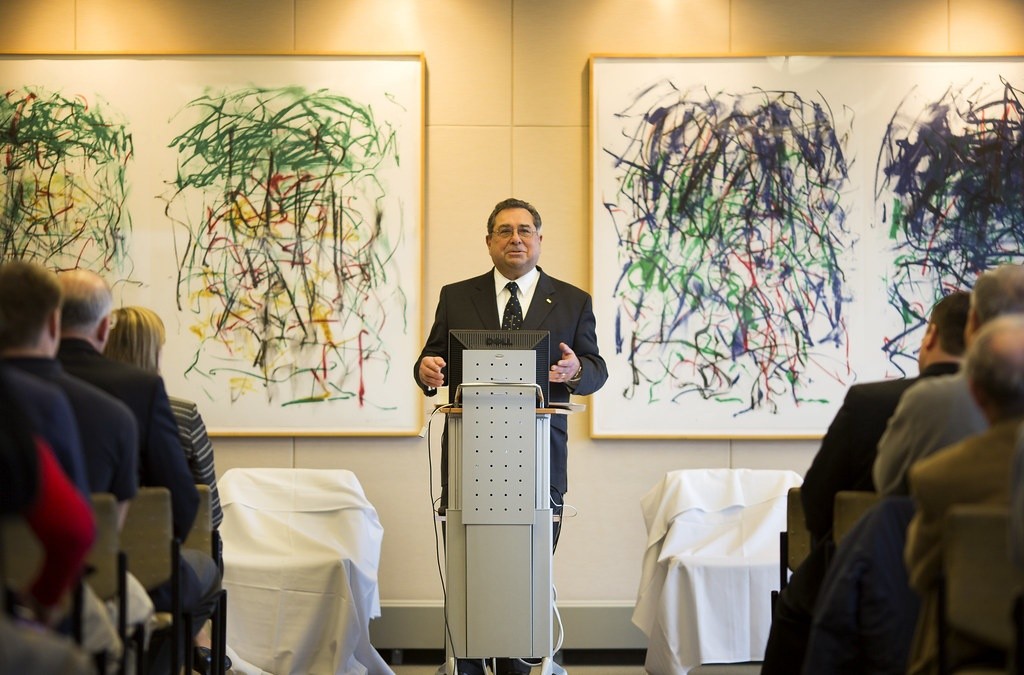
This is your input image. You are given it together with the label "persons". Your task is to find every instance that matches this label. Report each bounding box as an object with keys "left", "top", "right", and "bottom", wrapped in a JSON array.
[
  {"left": 760, "top": 290, "right": 971, "bottom": 556},
  {"left": 47, "top": 265, "right": 224, "bottom": 663},
  {"left": 412, "top": 195, "right": 610, "bottom": 675},
  {"left": 905, "top": 312, "right": 1024, "bottom": 673},
  {"left": 103, "top": 304, "right": 235, "bottom": 674},
  {"left": 0, "top": 258, "right": 139, "bottom": 534},
  {"left": 0, "top": 411, "right": 97, "bottom": 621},
  {"left": 870, "top": 261, "right": 1024, "bottom": 503}
]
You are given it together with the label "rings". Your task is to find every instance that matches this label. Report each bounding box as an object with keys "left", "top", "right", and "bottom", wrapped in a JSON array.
[{"left": 561, "top": 373, "right": 565, "bottom": 379}]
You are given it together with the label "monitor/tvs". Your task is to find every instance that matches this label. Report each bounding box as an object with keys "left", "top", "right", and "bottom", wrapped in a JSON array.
[{"left": 448, "top": 329, "right": 551, "bottom": 408}]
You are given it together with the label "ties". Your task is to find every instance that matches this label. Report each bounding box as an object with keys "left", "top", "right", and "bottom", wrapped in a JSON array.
[{"left": 502, "top": 282, "right": 523, "bottom": 331}]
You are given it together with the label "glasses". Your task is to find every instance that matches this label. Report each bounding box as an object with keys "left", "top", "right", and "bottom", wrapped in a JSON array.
[{"left": 490, "top": 227, "right": 540, "bottom": 239}]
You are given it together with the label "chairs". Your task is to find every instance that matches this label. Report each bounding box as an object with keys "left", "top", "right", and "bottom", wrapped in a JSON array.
[
  {"left": 181, "top": 484, "right": 225, "bottom": 675},
  {"left": 122, "top": 487, "right": 184, "bottom": 675},
  {"left": 936, "top": 505, "right": 1024, "bottom": 674},
  {"left": 6, "top": 517, "right": 42, "bottom": 603},
  {"left": 831, "top": 488, "right": 879, "bottom": 546},
  {"left": 85, "top": 491, "right": 128, "bottom": 675},
  {"left": 766, "top": 485, "right": 815, "bottom": 624}
]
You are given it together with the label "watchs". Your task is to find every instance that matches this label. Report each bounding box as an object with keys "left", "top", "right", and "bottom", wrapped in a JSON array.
[{"left": 573, "top": 365, "right": 582, "bottom": 379}]
